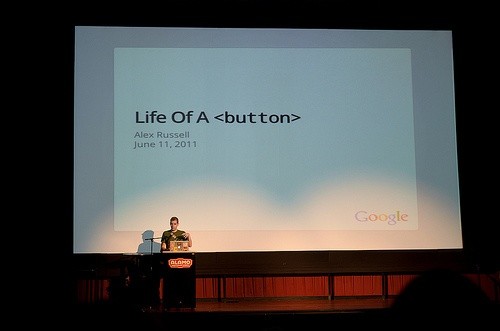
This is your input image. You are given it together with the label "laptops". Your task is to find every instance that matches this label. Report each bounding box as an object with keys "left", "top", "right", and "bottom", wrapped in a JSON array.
[{"left": 169, "top": 241, "right": 189, "bottom": 251}]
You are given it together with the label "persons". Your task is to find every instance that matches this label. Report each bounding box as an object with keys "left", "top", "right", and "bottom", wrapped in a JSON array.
[
  {"left": 159, "top": 217, "right": 193, "bottom": 251},
  {"left": 392, "top": 269, "right": 500, "bottom": 330}
]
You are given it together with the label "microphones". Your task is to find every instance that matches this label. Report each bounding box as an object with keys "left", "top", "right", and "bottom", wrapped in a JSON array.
[{"left": 165, "top": 232, "right": 173, "bottom": 243}]
